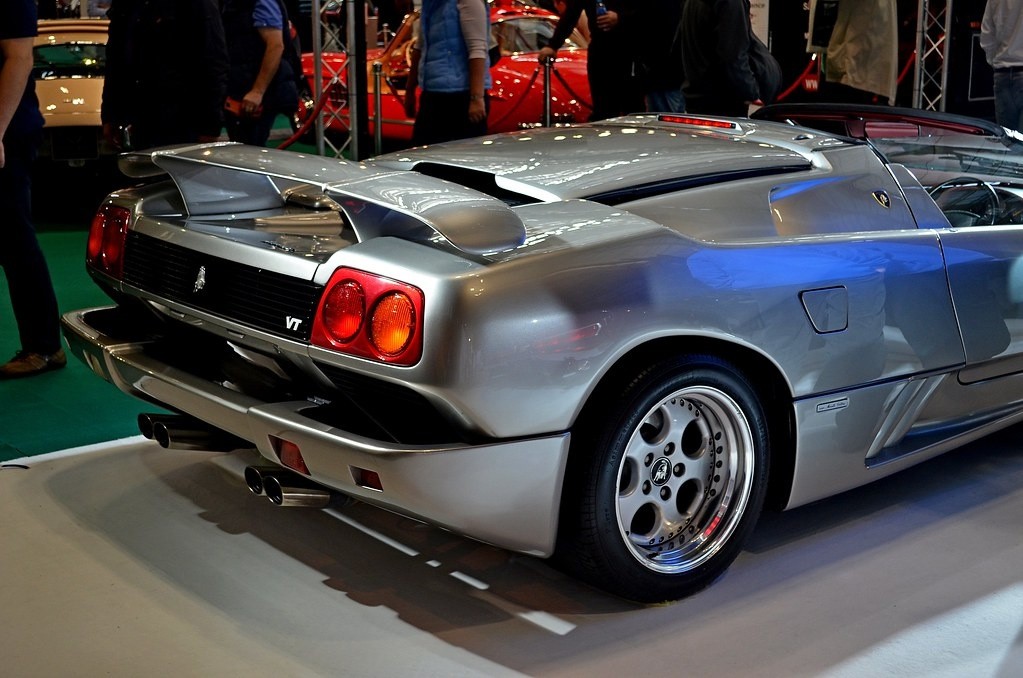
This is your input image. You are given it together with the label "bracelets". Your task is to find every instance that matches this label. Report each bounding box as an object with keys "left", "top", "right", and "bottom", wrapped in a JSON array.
[{"left": 471, "top": 95, "right": 484, "bottom": 100}]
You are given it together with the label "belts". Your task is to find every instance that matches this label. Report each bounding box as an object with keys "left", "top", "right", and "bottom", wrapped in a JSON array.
[{"left": 993, "top": 66, "right": 1023, "bottom": 74}]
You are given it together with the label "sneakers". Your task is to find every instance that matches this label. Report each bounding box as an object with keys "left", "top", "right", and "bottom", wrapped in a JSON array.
[{"left": 0, "top": 349, "right": 67, "bottom": 378}]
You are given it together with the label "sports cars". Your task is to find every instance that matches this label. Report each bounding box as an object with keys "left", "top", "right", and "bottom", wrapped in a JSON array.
[
  {"left": 15, "top": 40, "right": 108, "bottom": 151},
  {"left": 289, "top": 8, "right": 593, "bottom": 146},
  {"left": 58, "top": 105, "right": 1023, "bottom": 605}
]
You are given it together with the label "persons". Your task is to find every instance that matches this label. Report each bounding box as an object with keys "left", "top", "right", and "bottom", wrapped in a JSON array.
[
  {"left": 405, "top": 0, "right": 493, "bottom": 149},
  {"left": 0, "top": 0, "right": 68, "bottom": 379},
  {"left": 100, "top": 0, "right": 300, "bottom": 197},
  {"left": 538, "top": 0, "right": 897, "bottom": 122},
  {"left": 980, "top": 0, "right": 1023, "bottom": 134}
]
